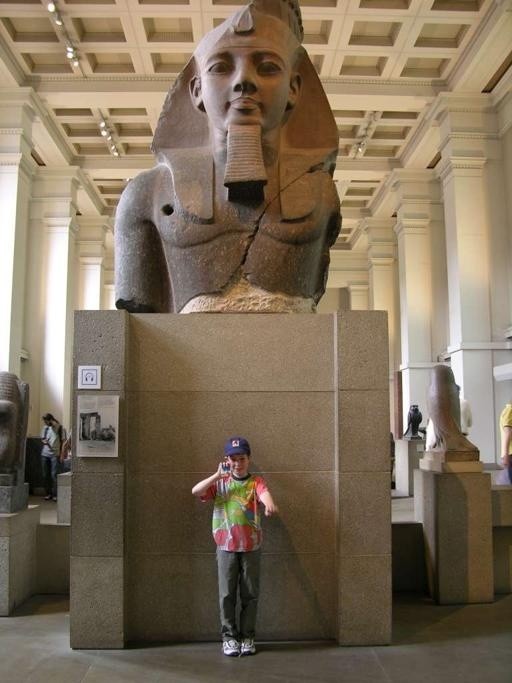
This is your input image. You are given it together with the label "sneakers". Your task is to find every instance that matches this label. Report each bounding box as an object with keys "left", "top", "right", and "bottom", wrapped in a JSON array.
[
  {"left": 44, "top": 494, "right": 57, "bottom": 502},
  {"left": 221, "top": 637, "right": 256, "bottom": 656}
]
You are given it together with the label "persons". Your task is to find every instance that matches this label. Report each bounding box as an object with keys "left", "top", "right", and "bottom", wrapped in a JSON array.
[
  {"left": 499, "top": 404, "right": 512, "bottom": 484},
  {"left": 191, "top": 438, "right": 279, "bottom": 657},
  {"left": 114, "top": 2, "right": 341, "bottom": 311},
  {"left": 41, "top": 414, "right": 72, "bottom": 501}
]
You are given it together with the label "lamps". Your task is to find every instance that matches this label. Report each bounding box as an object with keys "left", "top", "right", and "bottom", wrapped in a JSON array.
[
  {"left": 356, "top": 112, "right": 378, "bottom": 152},
  {"left": 48, "top": 1, "right": 79, "bottom": 67},
  {"left": 99, "top": 120, "right": 118, "bottom": 156}
]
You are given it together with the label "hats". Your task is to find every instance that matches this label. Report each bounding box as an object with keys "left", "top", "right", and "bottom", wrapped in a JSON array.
[
  {"left": 42, "top": 414, "right": 53, "bottom": 419},
  {"left": 224, "top": 436, "right": 250, "bottom": 456}
]
